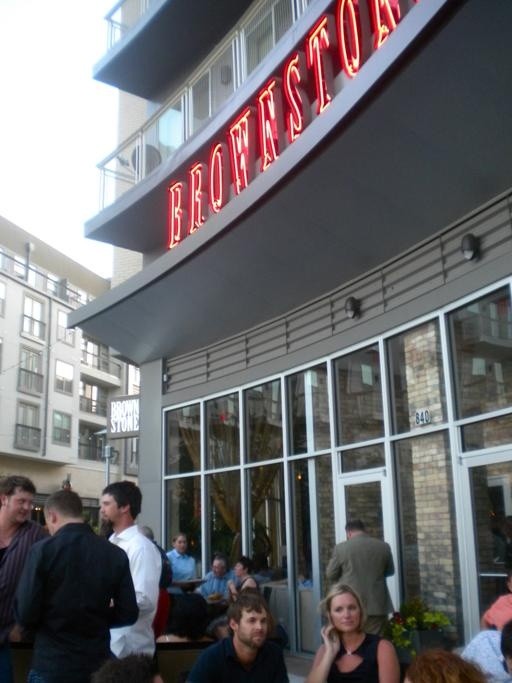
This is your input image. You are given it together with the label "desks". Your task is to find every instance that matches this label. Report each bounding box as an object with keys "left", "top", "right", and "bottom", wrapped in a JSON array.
[{"left": 204, "top": 598, "right": 229, "bottom": 619}]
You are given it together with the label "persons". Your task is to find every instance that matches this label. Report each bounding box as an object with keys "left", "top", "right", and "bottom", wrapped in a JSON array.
[
  {"left": 0, "top": 475, "right": 50, "bottom": 682},
  {"left": 14, "top": 489, "right": 140, "bottom": 683},
  {"left": 183, "top": 592, "right": 291, "bottom": 682},
  {"left": 304, "top": 583, "right": 401, "bottom": 683},
  {"left": 459, "top": 617, "right": 512, "bottom": 683},
  {"left": 139, "top": 525, "right": 258, "bottom": 640},
  {"left": 479, "top": 569, "right": 512, "bottom": 632},
  {"left": 323, "top": 519, "right": 395, "bottom": 637},
  {"left": 90, "top": 650, "right": 165, "bottom": 682},
  {"left": 98, "top": 481, "right": 163, "bottom": 659},
  {"left": 404, "top": 648, "right": 486, "bottom": 682}
]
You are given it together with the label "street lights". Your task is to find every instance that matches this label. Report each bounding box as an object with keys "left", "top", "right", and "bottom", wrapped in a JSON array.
[{"left": 93, "top": 428, "right": 114, "bottom": 487}]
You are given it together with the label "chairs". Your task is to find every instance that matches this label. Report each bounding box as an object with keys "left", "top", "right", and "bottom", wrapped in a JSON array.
[
  {"left": 155, "top": 639, "right": 216, "bottom": 682},
  {"left": 260, "top": 584, "right": 273, "bottom": 604}
]
